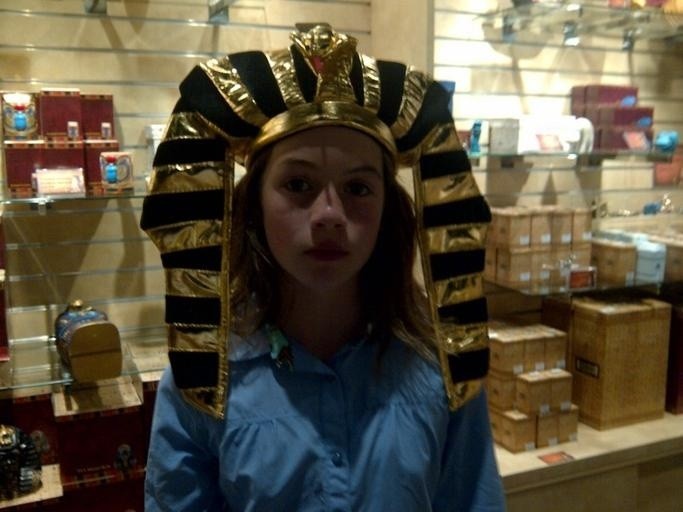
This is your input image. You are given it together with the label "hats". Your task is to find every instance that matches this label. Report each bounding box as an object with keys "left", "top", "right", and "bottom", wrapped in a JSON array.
[{"left": 141, "top": 25, "right": 493, "bottom": 422}]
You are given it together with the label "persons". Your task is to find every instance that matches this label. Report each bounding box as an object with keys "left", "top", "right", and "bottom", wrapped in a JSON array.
[{"left": 141, "top": 25, "right": 510, "bottom": 512}]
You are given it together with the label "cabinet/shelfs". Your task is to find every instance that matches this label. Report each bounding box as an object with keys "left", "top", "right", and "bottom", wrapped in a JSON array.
[
  {"left": 0, "top": 190, "right": 174, "bottom": 390},
  {"left": 469, "top": 2, "right": 682, "bottom": 298}
]
